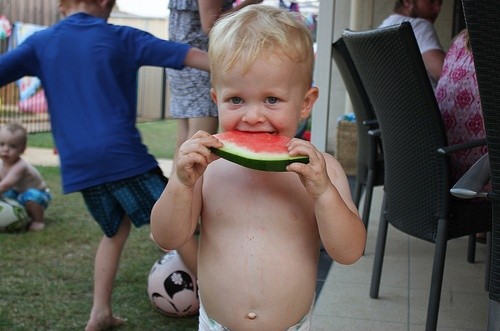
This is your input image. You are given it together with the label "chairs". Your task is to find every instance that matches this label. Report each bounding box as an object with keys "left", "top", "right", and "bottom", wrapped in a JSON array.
[{"left": 332, "top": 22, "right": 493, "bottom": 331}]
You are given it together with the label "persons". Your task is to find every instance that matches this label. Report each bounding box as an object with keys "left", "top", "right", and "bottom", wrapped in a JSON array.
[
  {"left": 434, "top": 27, "right": 496, "bottom": 245},
  {"left": 0, "top": 125, "right": 53, "bottom": 231},
  {"left": 167, "top": 0, "right": 263, "bottom": 149},
  {"left": 150, "top": 0, "right": 366, "bottom": 331},
  {"left": 1, "top": 0, "right": 210, "bottom": 330},
  {"left": 373, "top": 0, "right": 446, "bottom": 159}
]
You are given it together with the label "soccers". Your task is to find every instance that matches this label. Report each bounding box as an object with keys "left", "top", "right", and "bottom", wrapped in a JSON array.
[{"left": 147, "top": 250, "right": 198, "bottom": 316}]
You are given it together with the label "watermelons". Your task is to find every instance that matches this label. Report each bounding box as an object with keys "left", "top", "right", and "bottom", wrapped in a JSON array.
[{"left": 206, "top": 130, "right": 310, "bottom": 172}]
